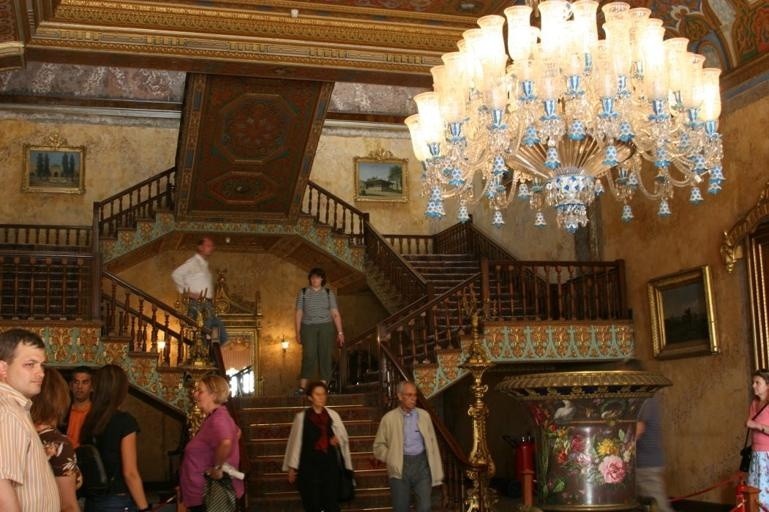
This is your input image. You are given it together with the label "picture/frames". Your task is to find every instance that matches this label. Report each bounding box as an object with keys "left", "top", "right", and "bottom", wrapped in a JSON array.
[
  {"left": 350, "top": 155, "right": 410, "bottom": 205},
  {"left": 20, "top": 141, "right": 87, "bottom": 194},
  {"left": 645, "top": 264, "right": 722, "bottom": 358}
]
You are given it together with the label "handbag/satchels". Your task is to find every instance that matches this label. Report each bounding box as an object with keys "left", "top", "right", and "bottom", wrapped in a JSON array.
[
  {"left": 739, "top": 444, "right": 751, "bottom": 472},
  {"left": 339, "top": 469, "right": 353, "bottom": 501}
]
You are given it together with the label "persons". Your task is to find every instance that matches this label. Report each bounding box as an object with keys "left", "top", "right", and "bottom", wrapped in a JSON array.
[
  {"left": 171, "top": 236, "right": 218, "bottom": 375},
  {"left": 28, "top": 364, "right": 84, "bottom": 512},
  {"left": 178, "top": 374, "right": 247, "bottom": 511},
  {"left": 292, "top": 268, "right": 345, "bottom": 398},
  {"left": 373, "top": 381, "right": 447, "bottom": 511},
  {"left": 66, "top": 365, "right": 93, "bottom": 450},
  {"left": 746, "top": 369, "right": 769, "bottom": 511},
  {"left": 281, "top": 380, "right": 355, "bottom": 512},
  {"left": 618, "top": 358, "right": 674, "bottom": 511},
  {"left": 75, "top": 364, "right": 157, "bottom": 511},
  {"left": 1, "top": 329, "right": 62, "bottom": 511}
]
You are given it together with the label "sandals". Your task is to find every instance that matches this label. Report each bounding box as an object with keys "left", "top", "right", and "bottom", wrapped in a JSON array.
[{"left": 294, "top": 386, "right": 306, "bottom": 396}]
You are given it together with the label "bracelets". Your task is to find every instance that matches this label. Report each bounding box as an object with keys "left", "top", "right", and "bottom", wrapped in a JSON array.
[
  {"left": 138, "top": 502, "right": 152, "bottom": 512},
  {"left": 337, "top": 331, "right": 345, "bottom": 336}
]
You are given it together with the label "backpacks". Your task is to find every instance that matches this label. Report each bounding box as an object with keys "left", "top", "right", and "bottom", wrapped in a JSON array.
[{"left": 74, "top": 444, "right": 121, "bottom": 498}]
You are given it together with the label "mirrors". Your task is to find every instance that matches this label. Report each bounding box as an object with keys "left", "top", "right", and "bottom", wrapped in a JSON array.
[{"left": 182, "top": 319, "right": 258, "bottom": 400}]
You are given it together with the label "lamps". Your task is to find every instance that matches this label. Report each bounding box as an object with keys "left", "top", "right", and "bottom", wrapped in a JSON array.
[
  {"left": 402, "top": 1, "right": 724, "bottom": 231},
  {"left": 279, "top": 338, "right": 288, "bottom": 350}
]
